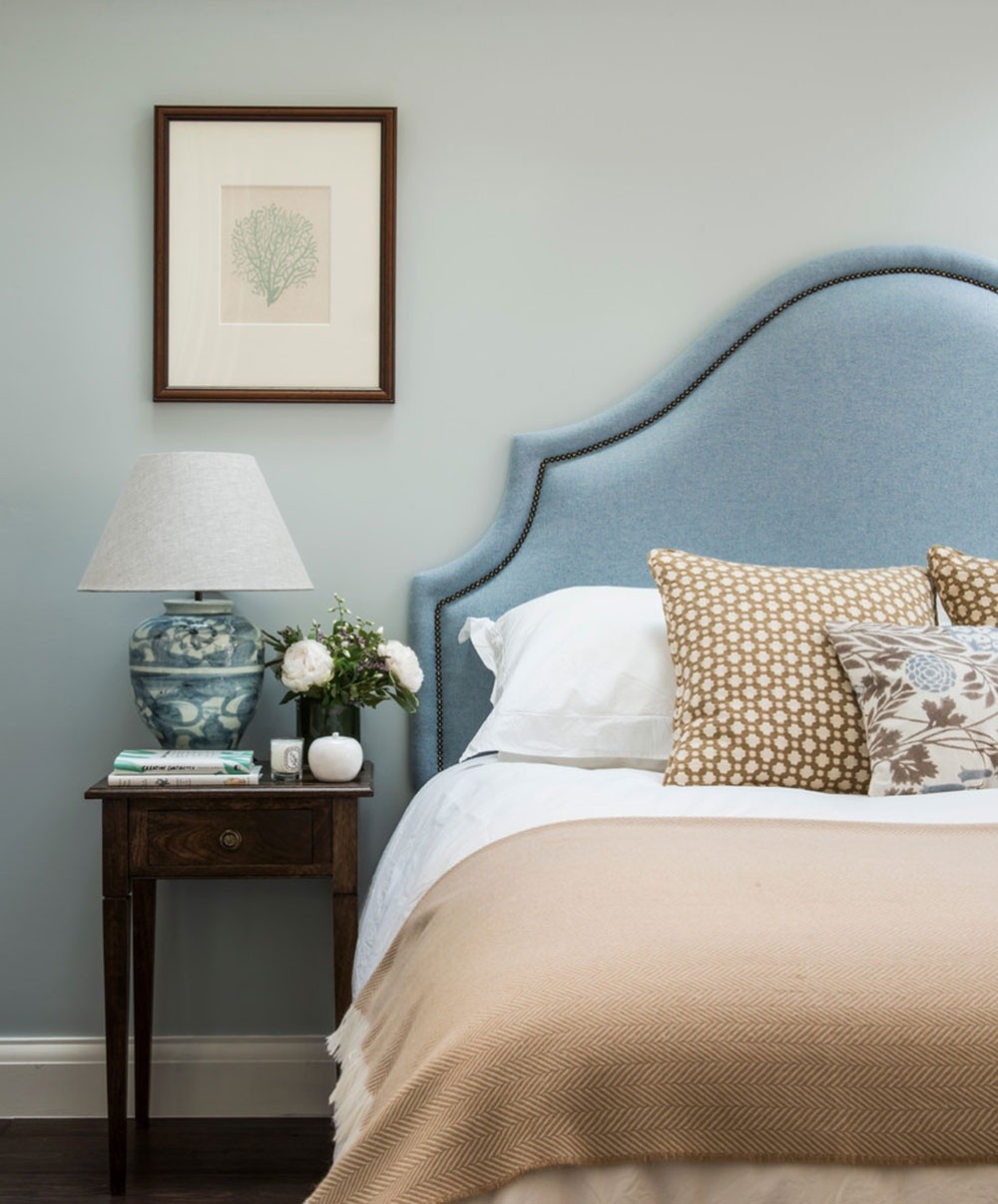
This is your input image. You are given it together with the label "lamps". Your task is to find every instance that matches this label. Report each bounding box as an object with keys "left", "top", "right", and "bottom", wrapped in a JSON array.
[{"left": 75, "top": 448, "right": 313, "bottom": 751}]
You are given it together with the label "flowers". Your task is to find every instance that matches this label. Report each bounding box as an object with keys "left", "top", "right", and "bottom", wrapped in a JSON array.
[{"left": 260, "top": 596, "right": 424, "bottom": 717}]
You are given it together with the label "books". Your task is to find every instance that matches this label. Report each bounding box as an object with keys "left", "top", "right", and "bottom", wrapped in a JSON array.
[
  {"left": 114, "top": 749, "right": 254, "bottom": 774},
  {"left": 107, "top": 765, "right": 262, "bottom": 787}
]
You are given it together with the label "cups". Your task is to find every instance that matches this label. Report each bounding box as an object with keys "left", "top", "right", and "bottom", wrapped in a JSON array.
[{"left": 271, "top": 737, "right": 304, "bottom": 783}]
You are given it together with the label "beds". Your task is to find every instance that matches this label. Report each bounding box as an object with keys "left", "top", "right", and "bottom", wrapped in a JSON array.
[{"left": 298, "top": 250, "right": 998, "bottom": 1204}]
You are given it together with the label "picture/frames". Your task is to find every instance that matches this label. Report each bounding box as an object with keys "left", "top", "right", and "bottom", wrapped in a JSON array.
[{"left": 155, "top": 104, "right": 398, "bottom": 404}]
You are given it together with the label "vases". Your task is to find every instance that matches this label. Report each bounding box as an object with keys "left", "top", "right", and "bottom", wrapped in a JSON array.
[{"left": 293, "top": 697, "right": 362, "bottom": 755}]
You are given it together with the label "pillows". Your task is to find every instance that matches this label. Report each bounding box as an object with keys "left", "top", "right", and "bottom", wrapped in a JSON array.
[
  {"left": 826, "top": 620, "right": 998, "bottom": 798},
  {"left": 455, "top": 583, "right": 678, "bottom": 769},
  {"left": 647, "top": 548, "right": 936, "bottom": 794},
  {"left": 927, "top": 543, "right": 998, "bottom": 628}
]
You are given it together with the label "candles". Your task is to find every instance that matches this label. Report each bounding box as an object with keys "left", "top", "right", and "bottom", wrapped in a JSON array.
[{"left": 265, "top": 737, "right": 304, "bottom": 782}]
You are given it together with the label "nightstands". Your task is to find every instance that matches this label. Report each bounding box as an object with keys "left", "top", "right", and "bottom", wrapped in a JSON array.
[{"left": 82, "top": 761, "right": 373, "bottom": 1197}]
